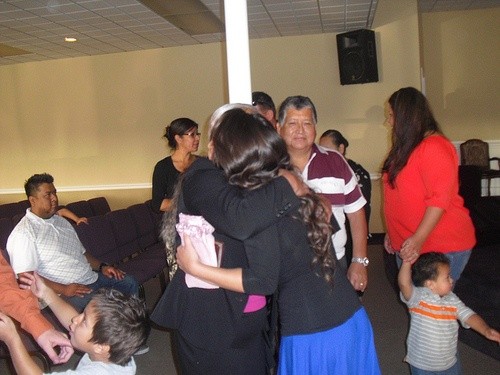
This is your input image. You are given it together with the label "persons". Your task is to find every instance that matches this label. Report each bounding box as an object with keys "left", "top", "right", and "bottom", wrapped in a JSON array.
[
  {"left": 0, "top": 270, "right": 151, "bottom": 375},
  {"left": 381, "top": 86, "right": 477, "bottom": 292},
  {"left": 56, "top": 208, "right": 89, "bottom": 226},
  {"left": 398, "top": 246, "right": 500, "bottom": 375},
  {"left": 150, "top": 90, "right": 382, "bottom": 375},
  {"left": 0, "top": 249, "right": 74, "bottom": 365},
  {"left": 6, "top": 173, "right": 150, "bottom": 356}
]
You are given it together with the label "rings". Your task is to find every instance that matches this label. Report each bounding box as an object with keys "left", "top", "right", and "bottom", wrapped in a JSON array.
[{"left": 359, "top": 282, "right": 364, "bottom": 286}]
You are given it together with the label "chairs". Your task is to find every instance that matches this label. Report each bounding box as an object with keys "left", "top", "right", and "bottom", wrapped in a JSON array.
[
  {"left": 460, "top": 139, "right": 500, "bottom": 198},
  {"left": 0, "top": 196, "right": 168, "bottom": 375}
]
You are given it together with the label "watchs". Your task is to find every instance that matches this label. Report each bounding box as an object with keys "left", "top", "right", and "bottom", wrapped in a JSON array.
[{"left": 351, "top": 257, "right": 370, "bottom": 267}]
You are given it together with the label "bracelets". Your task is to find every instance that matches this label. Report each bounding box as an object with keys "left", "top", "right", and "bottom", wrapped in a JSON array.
[{"left": 99, "top": 261, "right": 108, "bottom": 274}]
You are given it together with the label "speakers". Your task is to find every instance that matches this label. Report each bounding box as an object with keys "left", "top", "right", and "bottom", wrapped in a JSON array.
[{"left": 336, "top": 29, "right": 378, "bottom": 85}]
